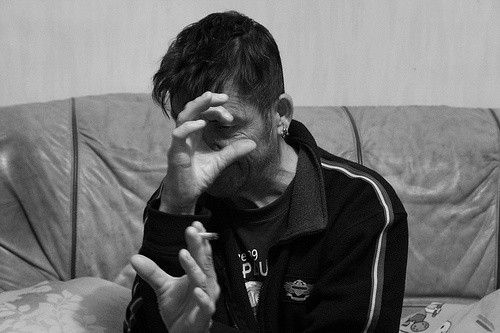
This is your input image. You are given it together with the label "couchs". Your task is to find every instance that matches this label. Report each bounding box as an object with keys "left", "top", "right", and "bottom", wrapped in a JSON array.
[{"left": 0, "top": 91, "right": 500, "bottom": 333}]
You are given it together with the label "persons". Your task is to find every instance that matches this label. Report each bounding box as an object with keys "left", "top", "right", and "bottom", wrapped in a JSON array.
[{"left": 123, "top": 9, "right": 408, "bottom": 333}]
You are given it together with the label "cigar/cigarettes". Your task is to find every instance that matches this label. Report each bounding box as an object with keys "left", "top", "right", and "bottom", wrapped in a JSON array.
[{"left": 198, "top": 232, "right": 220, "bottom": 241}]
